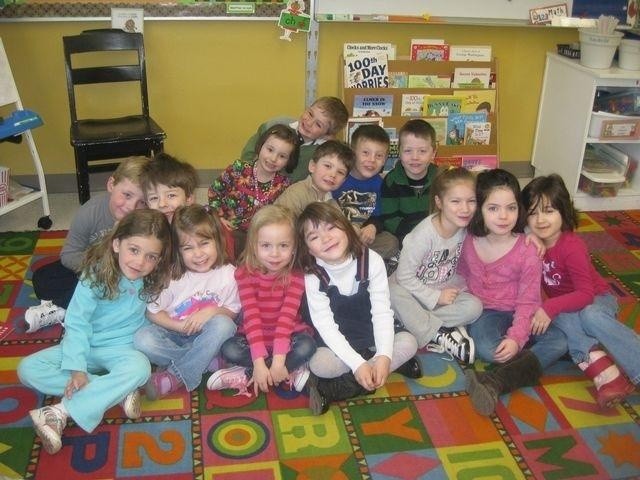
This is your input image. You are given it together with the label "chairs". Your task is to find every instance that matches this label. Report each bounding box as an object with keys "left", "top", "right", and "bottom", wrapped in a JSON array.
[{"left": 62, "top": 29, "right": 166, "bottom": 204}]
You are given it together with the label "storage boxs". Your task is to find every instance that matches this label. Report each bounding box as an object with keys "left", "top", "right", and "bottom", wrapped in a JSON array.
[{"left": 582, "top": 161, "right": 624, "bottom": 196}]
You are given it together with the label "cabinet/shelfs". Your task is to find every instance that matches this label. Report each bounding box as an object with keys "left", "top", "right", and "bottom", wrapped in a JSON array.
[{"left": 532, "top": 50, "right": 640, "bottom": 212}]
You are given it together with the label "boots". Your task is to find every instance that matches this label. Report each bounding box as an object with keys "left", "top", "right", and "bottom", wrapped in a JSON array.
[
  {"left": 309, "top": 370, "right": 376, "bottom": 416},
  {"left": 465, "top": 349, "right": 542, "bottom": 417},
  {"left": 394, "top": 357, "right": 424, "bottom": 379},
  {"left": 577, "top": 345, "right": 636, "bottom": 410}
]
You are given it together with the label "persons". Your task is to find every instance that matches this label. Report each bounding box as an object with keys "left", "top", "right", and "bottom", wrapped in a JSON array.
[
  {"left": 208, "top": 123, "right": 305, "bottom": 232},
  {"left": 273, "top": 140, "right": 355, "bottom": 218},
  {"left": 137, "top": 150, "right": 201, "bottom": 225},
  {"left": 295, "top": 201, "right": 423, "bottom": 416},
  {"left": 454, "top": 168, "right": 567, "bottom": 416},
  {"left": 24, "top": 155, "right": 148, "bottom": 334},
  {"left": 379, "top": 118, "right": 441, "bottom": 237},
  {"left": 133, "top": 203, "right": 241, "bottom": 402},
  {"left": 207, "top": 204, "right": 319, "bottom": 398},
  {"left": 385, "top": 165, "right": 484, "bottom": 368},
  {"left": 520, "top": 172, "right": 640, "bottom": 413},
  {"left": 331, "top": 124, "right": 400, "bottom": 260},
  {"left": 16, "top": 208, "right": 174, "bottom": 454},
  {"left": 240, "top": 96, "right": 349, "bottom": 185}
]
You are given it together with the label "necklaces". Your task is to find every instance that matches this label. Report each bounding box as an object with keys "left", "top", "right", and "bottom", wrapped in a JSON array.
[{"left": 251, "top": 170, "right": 280, "bottom": 211}]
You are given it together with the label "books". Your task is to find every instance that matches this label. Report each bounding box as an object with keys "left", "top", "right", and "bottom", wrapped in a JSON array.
[
  {"left": 410, "top": 43, "right": 449, "bottom": 64},
  {"left": 387, "top": 74, "right": 408, "bottom": 89},
  {"left": 111, "top": 7, "right": 144, "bottom": 34},
  {"left": 410, "top": 38, "right": 445, "bottom": 61},
  {"left": 343, "top": 49, "right": 390, "bottom": 89},
  {"left": 437, "top": 77, "right": 451, "bottom": 89},
  {"left": 401, "top": 94, "right": 424, "bottom": 117},
  {"left": 420, "top": 94, "right": 464, "bottom": 123},
  {"left": 446, "top": 111, "right": 487, "bottom": 146},
  {"left": 454, "top": 67, "right": 491, "bottom": 88},
  {"left": 420, "top": 118, "right": 447, "bottom": 146},
  {"left": 461, "top": 154, "right": 498, "bottom": 172},
  {"left": 453, "top": 90, "right": 497, "bottom": 113},
  {"left": 352, "top": 94, "right": 394, "bottom": 118},
  {"left": 432, "top": 156, "right": 463, "bottom": 169},
  {"left": 449, "top": 44, "right": 491, "bottom": 63},
  {"left": 408, "top": 74, "right": 437, "bottom": 89},
  {"left": 464, "top": 122, "right": 490, "bottom": 146},
  {"left": 346, "top": 118, "right": 384, "bottom": 144},
  {"left": 343, "top": 41, "right": 396, "bottom": 60}
]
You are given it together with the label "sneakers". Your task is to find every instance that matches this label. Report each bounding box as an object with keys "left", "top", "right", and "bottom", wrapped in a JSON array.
[
  {"left": 285, "top": 366, "right": 310, "bottom": 392},
  {"left": 119, "top": 391, "right": 143, "bottom": 420},
  {"left": 29, "top": 404, "right": 67, "bottom": 455},
  {"left": 146, "top": 371, "right": 179, "bottom": 401},
  {"left": 206, "top": 357, "right": 227, "bottom": 373},
  {"left": 24, "top": 300, "right": 66, "bottom": 334},
  {"left": 427, "top": 326, "right": 475, "bottom": 364},
  {"left": 206, "top": 366, "right": 252, "bottom": 398}
]
